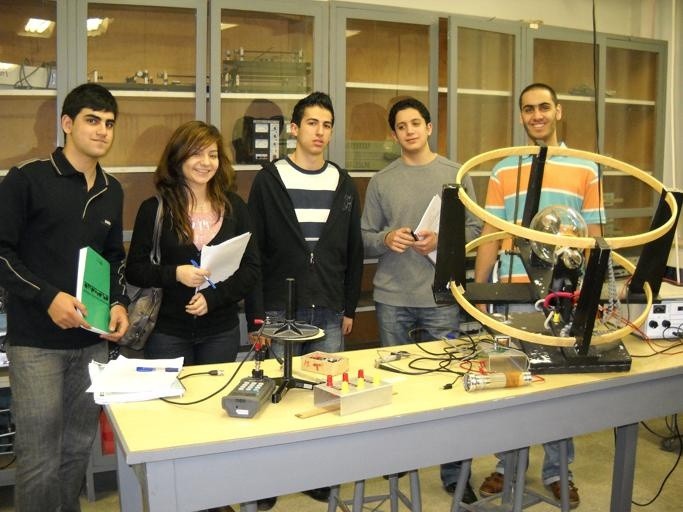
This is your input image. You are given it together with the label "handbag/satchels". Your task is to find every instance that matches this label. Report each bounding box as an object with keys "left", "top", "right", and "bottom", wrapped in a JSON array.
[{"left": 109, "top": 258, "right": 164, "bottom": 352}]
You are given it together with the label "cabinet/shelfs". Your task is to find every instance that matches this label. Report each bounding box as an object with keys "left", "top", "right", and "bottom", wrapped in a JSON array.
[
  {"left": 1, "top": 342, "right": 20, "bottom": 487},
  {"left": 524, "top": 18, "right": 670, "bottom": 257},
  {"left": 0, "top": 0, "right": 70, "bottom": 175},
  {"left": 70, "top": 1, "right": 336, "bottom": 256},
  {"left": 82, "top": 407, "right": 117, "bottom": 502},
  {"left": 332, "top": 0, "right": 522, "bottom": 348}
]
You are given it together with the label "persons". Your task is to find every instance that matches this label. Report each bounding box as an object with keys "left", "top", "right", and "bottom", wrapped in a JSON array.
[
  {"left": 473, "top": 83, "right": 606, "bottom": 510},
  {"left": 124, "top": 120, "right": 263, "bottom": 367},
  {"left": 360, "top": 98, "right": 486, "bottom": 504},
  {"left": 245, "top": 90, "right": 363, "bottom": 510},
  {"left": 0, "top": 85, "right": 130, "bottom": 512}
]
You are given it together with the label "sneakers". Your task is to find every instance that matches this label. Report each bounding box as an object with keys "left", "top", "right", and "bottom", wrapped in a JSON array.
[
  {"left": 257, "top": 497, "right": 276, "bottom": 510},
  {"left": 479, "top": 471, "right": 516, "bottom": 497},
  {"left": 446, "top": 481, "right": 478, "bottom": 506},
  {"left": 302, "top": 487, "right": 332, "bottom": 503},
  {"left": 383, "top": 472, "right": 407, "bottom": 480},
  {"left": 547, "top": 479, "right": 580, "bottom": 509}
]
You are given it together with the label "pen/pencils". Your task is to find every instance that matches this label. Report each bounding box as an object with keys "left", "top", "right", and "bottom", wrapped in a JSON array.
[
  {"left": 191, "top": 260, "right": 217, "bottom": 289},
  {"left": 136, "top": 367, "right": 184, "bottom": 372}
]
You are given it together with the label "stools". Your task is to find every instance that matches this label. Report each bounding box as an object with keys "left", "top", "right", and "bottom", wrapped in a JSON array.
[
  {"left": 328, "top": 468, "right": 421, "bottom": 512},
  {"left": 451, "top": 437, "right": 569, "bottom": 512}
]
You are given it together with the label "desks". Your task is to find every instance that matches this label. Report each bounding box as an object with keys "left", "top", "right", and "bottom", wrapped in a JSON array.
[{"left": 104, "top": 279, "right": 683, "bottom": 511}]
